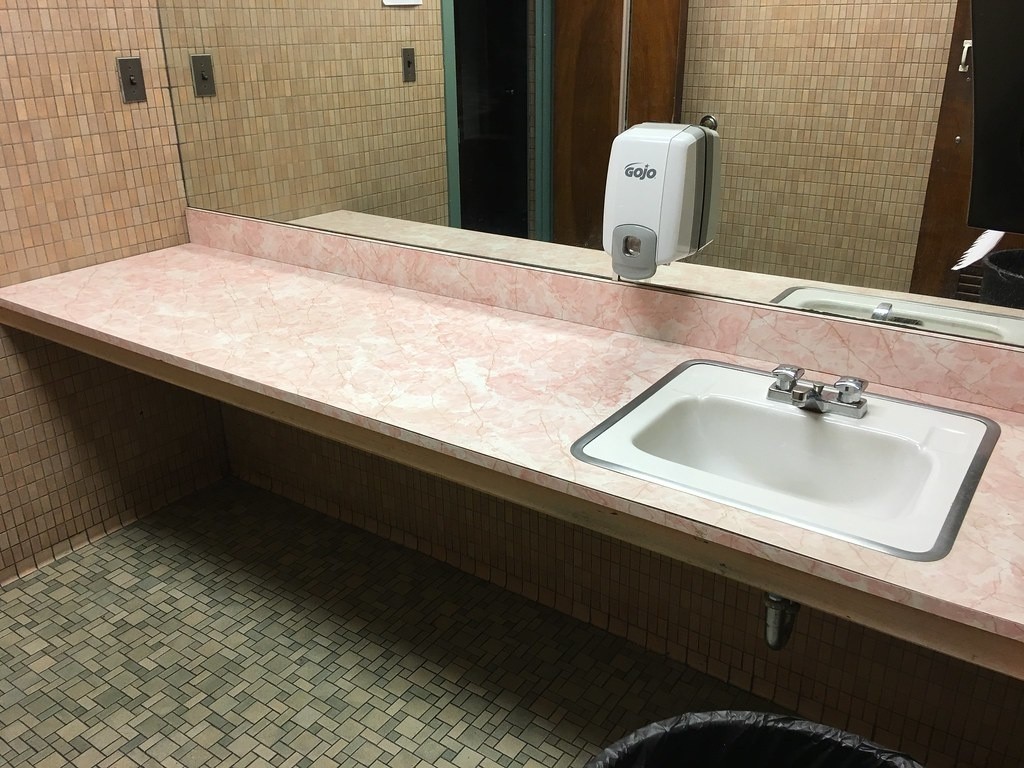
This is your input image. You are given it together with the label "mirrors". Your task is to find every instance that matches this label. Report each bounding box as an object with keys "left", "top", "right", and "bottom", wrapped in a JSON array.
[{"left": 156, "top": 2, "right": 1024, "bottom": 352}]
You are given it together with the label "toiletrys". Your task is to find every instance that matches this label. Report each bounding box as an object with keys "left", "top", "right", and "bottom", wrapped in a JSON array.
[
  {"left": 686, "top": 124, "right": 718, "bottom": 254},
  {"left": 600, "top": 122, "right": 709, "bottom": 282}
]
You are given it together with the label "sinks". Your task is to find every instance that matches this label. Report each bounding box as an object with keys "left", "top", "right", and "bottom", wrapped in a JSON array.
[
  {"left": 570, "top": 354, "right": 1005, "bottom": 570},
  {"left": 768, "top": 287, "right": 1024, "bottom": 356}
]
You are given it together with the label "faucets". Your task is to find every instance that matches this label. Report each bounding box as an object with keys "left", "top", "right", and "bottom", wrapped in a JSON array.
[
  {"left": 869, "top": 299, "right": 895, "bottom": 325},
  {"left": 790, "top": 380, "right": 832, "bottom": 413}
]
